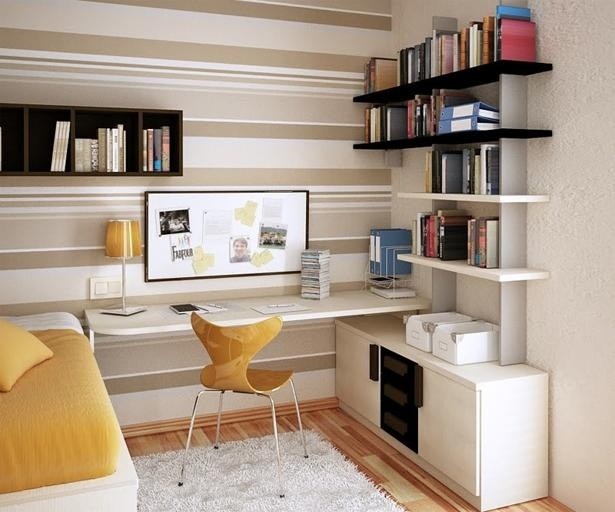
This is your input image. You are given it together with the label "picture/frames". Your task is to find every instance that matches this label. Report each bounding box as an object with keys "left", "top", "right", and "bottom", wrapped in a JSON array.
[{"left": 144, "top": 190, "right": 309, "bottom": 283}]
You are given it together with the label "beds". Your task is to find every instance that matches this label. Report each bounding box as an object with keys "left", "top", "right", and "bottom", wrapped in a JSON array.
[{"left": 0, "top": 312, "right": 139, "bottom": 512}]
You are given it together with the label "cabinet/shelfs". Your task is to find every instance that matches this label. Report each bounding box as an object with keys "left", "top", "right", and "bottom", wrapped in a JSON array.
[
  {"left": 397, "top": 192, "right": 550, "bottom": 282},
  {"left": 352, "top": 59, "right": 552, "bottom": 150},
  {"left": 0, "top": 102, "right": 183, "bottom": 176}
]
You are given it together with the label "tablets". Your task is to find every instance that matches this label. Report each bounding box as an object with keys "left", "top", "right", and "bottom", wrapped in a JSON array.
[{"left": 170, "top": 304, "right": 200, "bottom": 314}]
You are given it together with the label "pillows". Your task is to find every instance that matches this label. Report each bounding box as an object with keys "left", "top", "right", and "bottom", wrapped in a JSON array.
[{"left": 0, "top": 318, "right": 54, "bottom": 392}]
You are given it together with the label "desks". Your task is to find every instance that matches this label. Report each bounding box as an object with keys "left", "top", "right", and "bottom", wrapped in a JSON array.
[{"left": 85, "top": 288, "right": 433, "bottom": 380}]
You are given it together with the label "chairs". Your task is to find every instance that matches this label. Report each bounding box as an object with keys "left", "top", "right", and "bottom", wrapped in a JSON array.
[{"left": 178, "top": 312, "right": 308, "bottom": 498}]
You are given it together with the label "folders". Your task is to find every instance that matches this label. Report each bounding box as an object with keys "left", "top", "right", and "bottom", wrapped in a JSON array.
[
  {"left": 369, "top": 229, "right": 412, "bottom": 276},
  {"left": 439, "top": 102, "right": 499, "bottom": 135}
]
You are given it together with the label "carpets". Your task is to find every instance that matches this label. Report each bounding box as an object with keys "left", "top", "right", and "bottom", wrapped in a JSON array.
[{"left": 131, "top": 428, "right": 411, "bottom": 512}]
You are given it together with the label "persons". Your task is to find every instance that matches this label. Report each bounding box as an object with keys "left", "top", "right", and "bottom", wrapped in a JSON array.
[
  {"left": 170, "top": 218, "right": 186, "bottom": 231},
  {"left": 230, "top": 237, "right": 251, "bottom": 262}
]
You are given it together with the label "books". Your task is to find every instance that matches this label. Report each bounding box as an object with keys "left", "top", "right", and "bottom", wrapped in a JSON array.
[
  {"left": 411, "top": 208, "right": 499, "bottom": 269},
  {"left": 49, "top": 121, "right": 171, "bottom": 172},
  {"left": 364, "top": 5, "right": 538, "bottom": 143},
  {"left": 300, "top": 248, "right": 331, "bottom": 300},
  {"left": 424, "top": 144, "right": 499, "bottom": 195}
]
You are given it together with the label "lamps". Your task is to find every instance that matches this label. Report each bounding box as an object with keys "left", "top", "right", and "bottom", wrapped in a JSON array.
[{"left": 100, "top": 220, "right": 146, "bottom": 316}]
